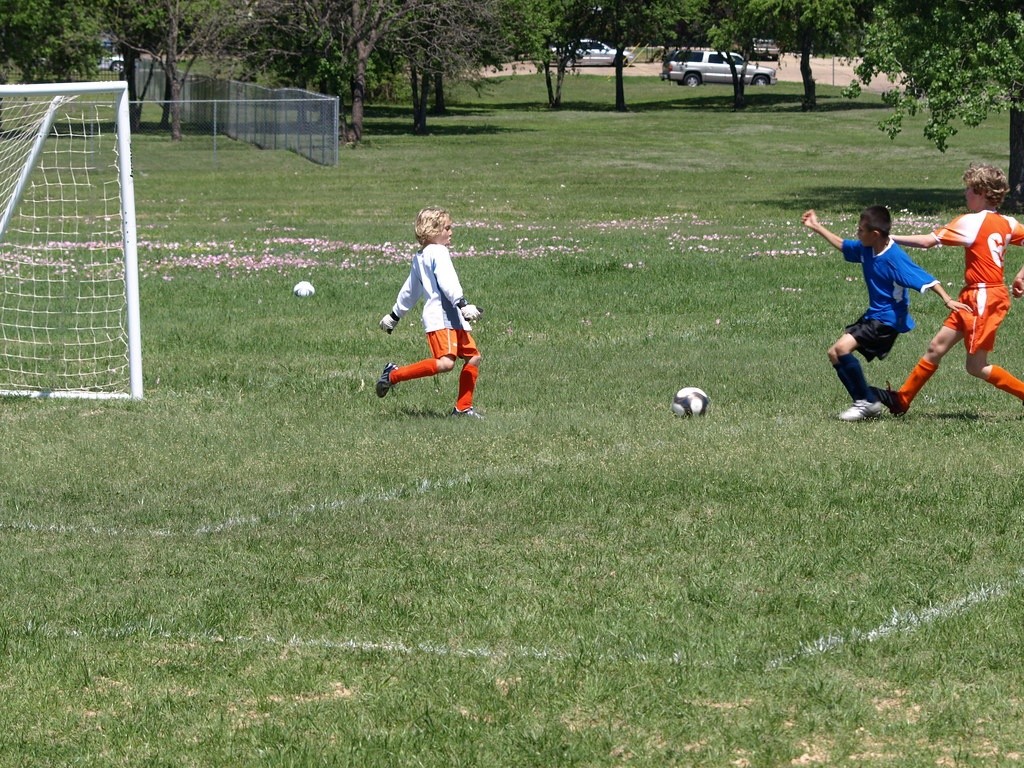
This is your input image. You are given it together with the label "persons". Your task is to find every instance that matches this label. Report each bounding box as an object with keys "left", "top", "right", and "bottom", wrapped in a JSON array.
[
  {"left": 377, "top": 208, "right": 487, "bottom": 420},
  {"left": 800, "top": 204, "right": 973, "bottom": 423},
  {"left": 868, "top": 162, "right": 1024, "bottom": 418}
]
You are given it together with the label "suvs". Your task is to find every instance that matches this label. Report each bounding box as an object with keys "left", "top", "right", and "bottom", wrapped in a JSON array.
[{"left": 659, "top": 49, "right": 778, "bottom": 88}]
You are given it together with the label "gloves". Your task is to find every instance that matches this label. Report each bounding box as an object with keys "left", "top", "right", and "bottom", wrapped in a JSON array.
[
  {"left": 460, "top": 302, "right": 484, "bottom": 324},
  {"left": 379, "top": 312, "right": 400, "bottom": 335}
]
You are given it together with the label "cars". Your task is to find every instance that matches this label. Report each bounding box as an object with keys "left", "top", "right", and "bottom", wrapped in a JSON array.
[
  {"left": 96, "top": 55, "right": 125, "bottom": 75},
  {"left": 740, "top": 38, "right": 779, "bottom": 61},
  {"left": 532, "top": 39, "right": 635, "bottom": 66}
]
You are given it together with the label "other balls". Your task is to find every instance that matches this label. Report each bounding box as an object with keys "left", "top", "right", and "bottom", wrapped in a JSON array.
[{"left": 292, "top": 278, "right": 315, "bottom": 297}]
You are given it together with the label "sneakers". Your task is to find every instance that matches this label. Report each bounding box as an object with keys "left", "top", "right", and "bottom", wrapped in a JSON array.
[
  {"left": 451, "top": 407, "right": 485, "bottom": 420},
  {"left": 840, "top": 398, "right": 882, "bottom": 421},
  {"left": 376, "top": 362, "right": 399, "bottom": 398},
  {"left": 872, "top": 381, "right": 910, "bottom": 417}
]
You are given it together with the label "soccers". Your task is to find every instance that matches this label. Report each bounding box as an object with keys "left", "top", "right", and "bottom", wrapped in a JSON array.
[{"left": 673, "top": 386, "right": 709, "bottom": 419}]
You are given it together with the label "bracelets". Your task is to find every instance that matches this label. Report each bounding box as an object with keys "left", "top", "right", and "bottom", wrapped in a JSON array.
[{"left": 945, "top": 299, "right": 953, "bottom": 306}]
